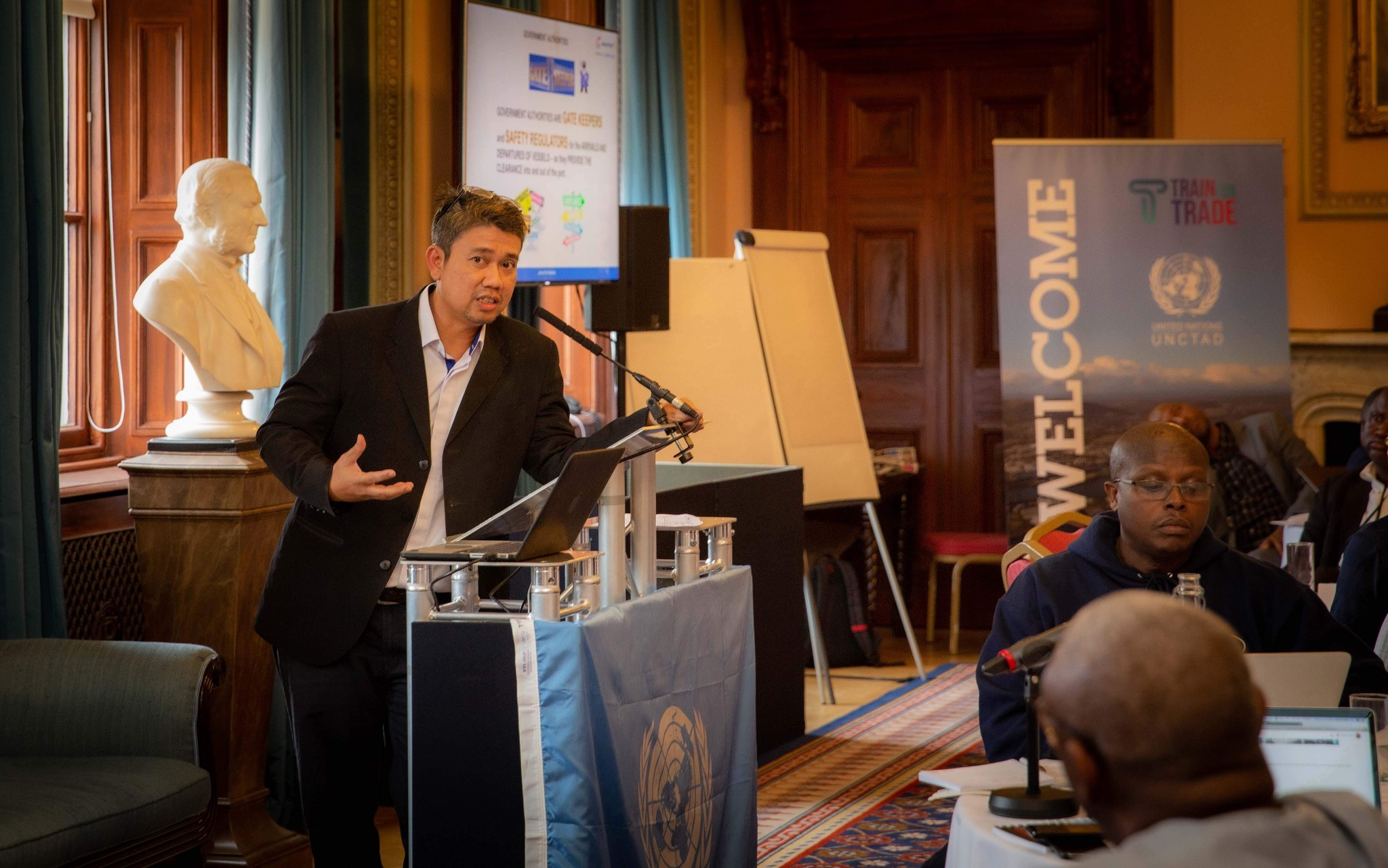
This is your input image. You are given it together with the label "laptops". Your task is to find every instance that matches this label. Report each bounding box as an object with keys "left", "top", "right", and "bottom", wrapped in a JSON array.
[
  {"left": 400, "top": 446, "right": 628, "bottom": 564},
  {"left": 1239, "top": 652, "right": 1382, "bottom": 816}
]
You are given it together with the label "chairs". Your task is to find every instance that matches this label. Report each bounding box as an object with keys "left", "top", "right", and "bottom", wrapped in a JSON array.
[{"left": 921, "top": 529, "right": 1009, "bottom": 655}]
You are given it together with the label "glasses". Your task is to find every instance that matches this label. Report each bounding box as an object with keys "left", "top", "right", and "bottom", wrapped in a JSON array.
[
  {"left": 1035, "top": 697, "right": 1112, "bottom": 791},
  {"left": 1113, "top": 479, "right": 1215, "bottom": 502}
]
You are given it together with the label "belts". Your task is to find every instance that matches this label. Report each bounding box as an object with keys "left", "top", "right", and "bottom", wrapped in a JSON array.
[{"left": 377, "top": 586, "right": 452, "bottom": 605}]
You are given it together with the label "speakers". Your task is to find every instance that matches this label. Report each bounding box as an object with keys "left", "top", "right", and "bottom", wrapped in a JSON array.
[{"left": 583, "top": 205, "right": 671, "bottom": 332}]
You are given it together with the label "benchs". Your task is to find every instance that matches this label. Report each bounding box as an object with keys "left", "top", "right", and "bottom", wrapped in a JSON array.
[{"left": 0, "top": 638, "right": 225, "bottom": 868}]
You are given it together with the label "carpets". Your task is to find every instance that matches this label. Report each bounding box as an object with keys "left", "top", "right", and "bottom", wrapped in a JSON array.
[{"left": 756, "top": 660, "right": 989, "bottom": 868}]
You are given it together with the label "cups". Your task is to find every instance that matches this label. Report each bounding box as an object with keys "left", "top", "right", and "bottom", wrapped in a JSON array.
[{"left": 1349, "top": 693, "right": 1388, "bottom": 782}]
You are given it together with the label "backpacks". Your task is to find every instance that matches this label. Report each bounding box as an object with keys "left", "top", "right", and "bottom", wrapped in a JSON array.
[{"left": 804, "top": 551, "right": 905, "bottom": 669}]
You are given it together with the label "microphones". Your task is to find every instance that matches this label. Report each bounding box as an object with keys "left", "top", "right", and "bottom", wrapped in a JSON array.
[
  {"left": 531, "top": 305, "right": 699, "bottom": 420},
  {"left": 981, "top": 623, "right": 1070, "bottom": 678}
]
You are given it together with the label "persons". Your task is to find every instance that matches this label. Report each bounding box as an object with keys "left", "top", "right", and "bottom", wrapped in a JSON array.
[
  {"left": 1034, "top": 588, "right": 1388, "bottom": 868},
  {"left": 1282, "top": 385, "right": 1388, "bottom": 583},
  {"left": 132, "top": 158, "right": 285, "bottom": 392},
  {"left": 1146, "top": 402, "right": 1322, "bottom": 569},
  {"left": 976, "top": 421, "right": 1388, "bottom": 762},
  {"left": 253, "top": 188, "right": 704, "bottom": 868}
]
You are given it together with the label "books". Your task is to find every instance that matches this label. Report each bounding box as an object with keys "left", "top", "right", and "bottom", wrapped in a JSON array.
[{"left": 918, "top": 758, "right": 1054, "bottom": 794}]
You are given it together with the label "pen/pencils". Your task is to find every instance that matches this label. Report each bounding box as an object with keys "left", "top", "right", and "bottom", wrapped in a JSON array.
[{"left": 1019, "top": 757, "right": 1047, "bottom": 773}]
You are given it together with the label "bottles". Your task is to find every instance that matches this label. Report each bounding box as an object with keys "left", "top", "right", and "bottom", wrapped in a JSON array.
[{"left": 1176, "top": 572, "right": 1204, "bottom": 610}]
